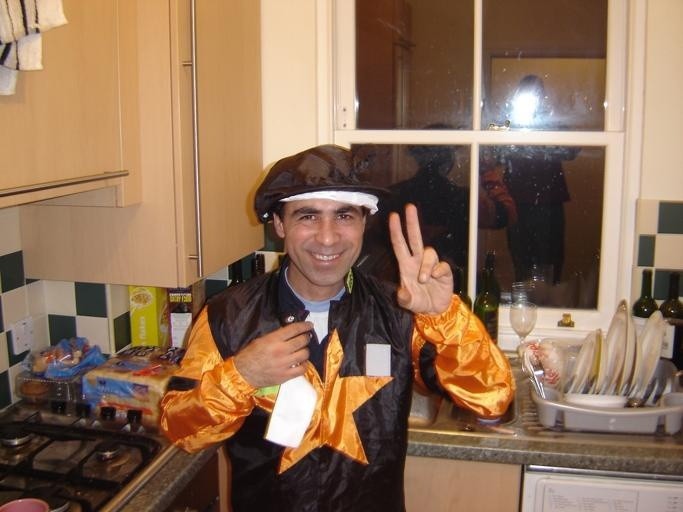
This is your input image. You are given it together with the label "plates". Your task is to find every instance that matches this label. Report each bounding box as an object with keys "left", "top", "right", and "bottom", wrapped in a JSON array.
[{"left": 567, "top": 296, "right": 664, "bottom": 403}]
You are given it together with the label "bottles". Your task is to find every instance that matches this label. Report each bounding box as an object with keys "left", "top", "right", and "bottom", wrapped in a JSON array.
[
  {"left": 479, "top": 250, "right": 500, "bottom": 300},
  {"left": 658, "top": 270, "right": 682, "bottom": 372},
  {"left": 456, "top": 269, "right": 471, "bottom": 310},
  {"left": 632, "top": 266, "right": 658, "bottom": 338},
  {"left": 476, "top": 267, "right": 497, "bottom": 344}
]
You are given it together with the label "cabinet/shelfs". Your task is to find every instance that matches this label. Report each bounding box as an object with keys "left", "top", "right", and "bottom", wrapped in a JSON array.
[
  {"left": 20, "top": 0, "right": 265, "bottom": 289},
  {"left": 519, "top": 465, "right": 682, "bottom": 512},
  {"left": 0, "top": 0, "right": 139, "bottom": 209}
]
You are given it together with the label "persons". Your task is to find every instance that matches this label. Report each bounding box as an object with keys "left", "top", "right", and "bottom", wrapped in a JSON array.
[
  {"left": 158, "top": 142, "right": 516, "bottom": 508},
  {"left": 385, "top": 127, "right": 513, "bottom": 294},
  {"left": 502, "top": 76, "right": 581, "bottom": 284}
]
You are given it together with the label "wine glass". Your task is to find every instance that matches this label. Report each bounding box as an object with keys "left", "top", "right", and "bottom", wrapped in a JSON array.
[{"left": 509, "top": 300, "right": 537, "bottom": 366}]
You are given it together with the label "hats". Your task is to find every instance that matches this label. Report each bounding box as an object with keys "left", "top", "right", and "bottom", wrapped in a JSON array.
[
  {"left": 253, "top": 144, "right": 391, "bottom": 223},
  {"left": 403, "top": 123, "right": 463, "bottom": 155}
]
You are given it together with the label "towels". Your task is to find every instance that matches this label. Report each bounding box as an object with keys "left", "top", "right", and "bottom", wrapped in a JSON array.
[{"left": 0, "top": 0, "right": 68, "bottom": 98}]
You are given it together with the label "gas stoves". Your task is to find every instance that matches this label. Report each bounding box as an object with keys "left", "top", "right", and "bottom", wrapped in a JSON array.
[{"left": 0, "top": 394, "right": 187, "bottom": 512}]
